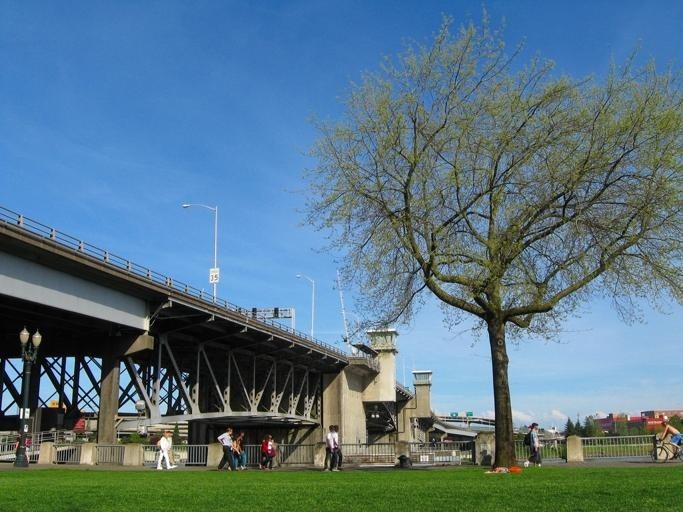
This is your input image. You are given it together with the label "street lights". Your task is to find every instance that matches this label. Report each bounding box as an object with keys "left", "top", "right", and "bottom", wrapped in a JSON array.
[
  {"left": 179, "top": 201, "right": 220, "bottom": 303},
  {"left": 10, "top": 327, "right": 45, "bottom": 468},
  {"left": 134, "top": 399, "right": 147, "bottom": 437},
  {"left": 296, "top": 273, "right": 316, "bottom": 342}
]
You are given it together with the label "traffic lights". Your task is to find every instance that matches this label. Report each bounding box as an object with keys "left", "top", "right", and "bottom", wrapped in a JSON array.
[
  {"left": 252, "top": 307, "right": 257, "bottom": 321},
  {"left": 274, "top": 308, "right": 279, "bottom": 318}
]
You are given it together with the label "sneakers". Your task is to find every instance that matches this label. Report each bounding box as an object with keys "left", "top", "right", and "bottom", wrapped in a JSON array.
[
  {"left": 227, "top": 465, "right": 248, "bottom": 472},
  {"left": 323, "top": 467, "right": 344, "bottom": 472}
]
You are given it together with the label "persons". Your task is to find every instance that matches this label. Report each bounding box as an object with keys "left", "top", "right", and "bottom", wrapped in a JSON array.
[
  {"left": 523, "top": 423, "right": 542, "bottom": 467},
  {"left": 25, "top": 434, "right": 30, "bottom": 464},
  {"left": 15, "top": 432, "right": 22, "bottom": 449},
  {"left": 217, "top": 427, "right": 274, "bottom": 471},
  {"left": 156, "top": 430, "right": 178, "bottom": 470},
  {"left": 323, "top": 425, "right": 339, "bottom": 471},
  {"left": 329, "top": 425, "right": 344, "bottom": 470},
  {"left": 659, "top": 421, "right": 681, "bottom": 459}
]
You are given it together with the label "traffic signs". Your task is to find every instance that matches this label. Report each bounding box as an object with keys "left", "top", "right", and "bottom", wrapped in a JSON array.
[
  {"left": 208, "top": 268, "right": 220, "bottom": 284},
  {"left": 466, "top": 412, "right": 473, "bottom": 417},
  {"left": 449, "top": 412, "right": 459, "bottom": 417}
]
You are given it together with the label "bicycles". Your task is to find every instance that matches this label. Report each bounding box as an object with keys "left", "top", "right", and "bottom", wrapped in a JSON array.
[{"left": 651, "top": 439, "right": 683, "bottom": 463}]
